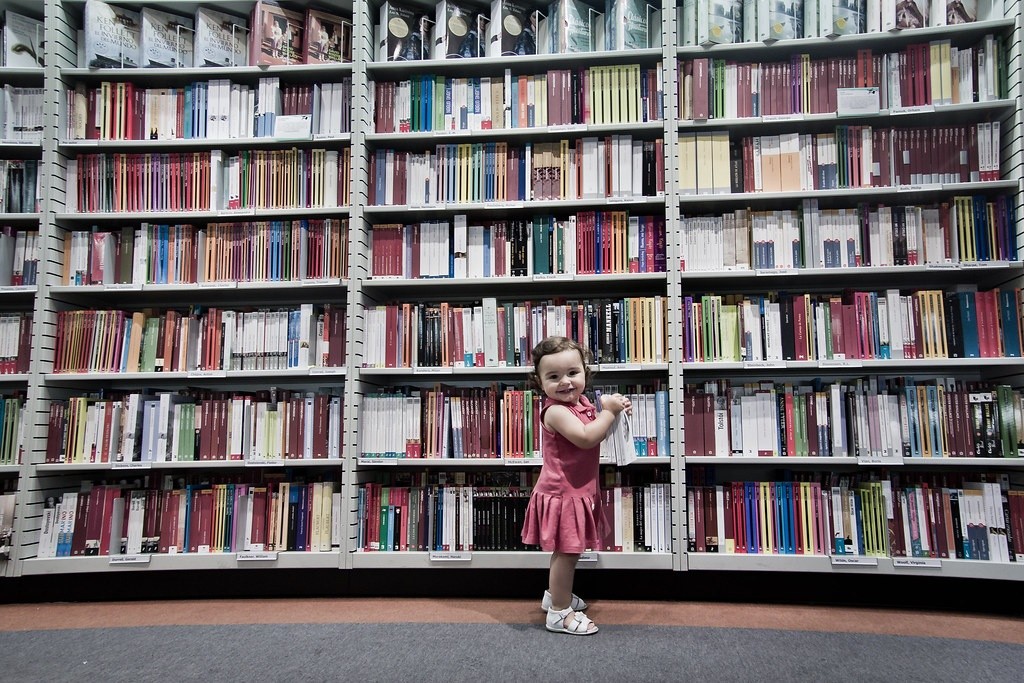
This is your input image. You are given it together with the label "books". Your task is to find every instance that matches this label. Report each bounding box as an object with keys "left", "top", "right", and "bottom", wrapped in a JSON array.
[
  {"left": 0, "top": 6, "right": 47, "bottom": 561},
  {"left": 677, "top": 1, "right": 1024, "bottom": 564},
  {"left": 355, "top": 1, "right": 673, "bottom": 556},
  {"left": 37, "top": 0, "right": 353, "bottom": 562}
]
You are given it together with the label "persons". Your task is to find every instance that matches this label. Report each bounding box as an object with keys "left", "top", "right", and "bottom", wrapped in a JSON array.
[{"left": 519, "top": 337, "right": 633, "bottom": 636}]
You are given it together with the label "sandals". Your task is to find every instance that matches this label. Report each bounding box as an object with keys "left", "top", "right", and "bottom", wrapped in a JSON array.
[
  {"left": 540, "top": 589, "right": 588, "bottom": 610},
  {"left": 545, "top": 606, "right": 598, "bottom": 635}
]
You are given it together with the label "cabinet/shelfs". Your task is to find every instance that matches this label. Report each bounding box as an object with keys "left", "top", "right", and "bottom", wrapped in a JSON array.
[
  {"left": 345, "top": 0, "right": 681, "bottom": 569},
  {"left": 670, "top": 0, "right": 1024, "bottom": 582},
  {"left": 13, "top": 0, "right": 359, "bottom": 577},
  {"left": 0, "top": 0, "right": 52, "bottom": 576}
]
